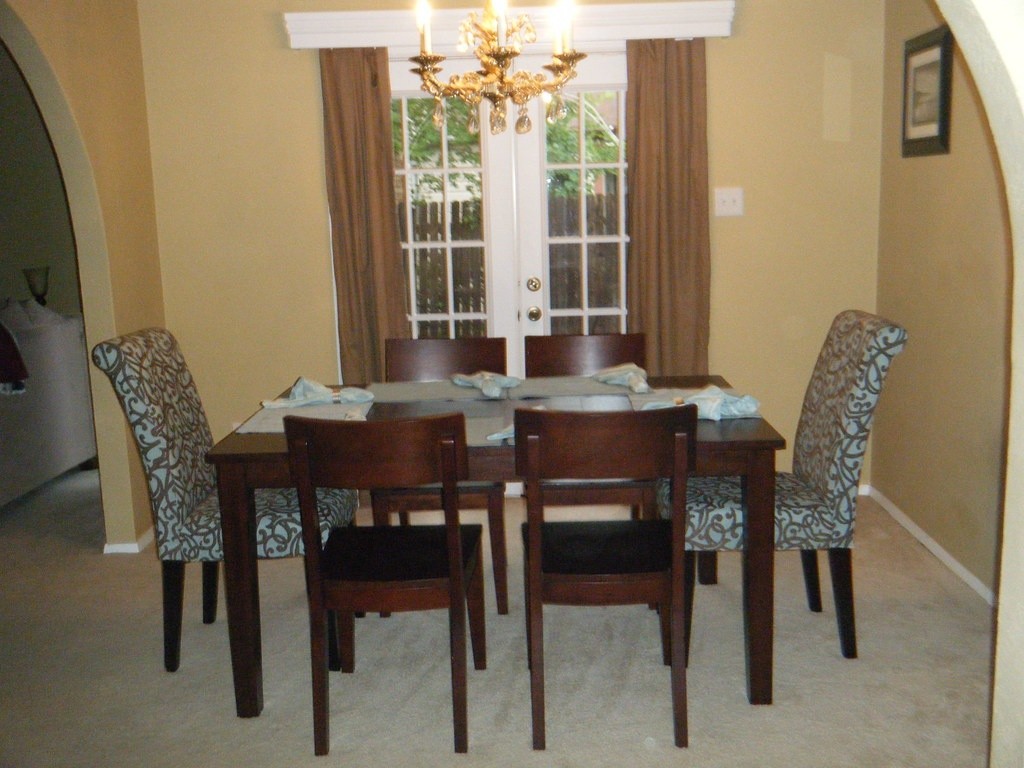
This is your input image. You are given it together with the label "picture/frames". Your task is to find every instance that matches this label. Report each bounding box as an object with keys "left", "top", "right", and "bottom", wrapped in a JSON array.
[{"left": 901, "top": 25, "right": 955, "bottom": 157}]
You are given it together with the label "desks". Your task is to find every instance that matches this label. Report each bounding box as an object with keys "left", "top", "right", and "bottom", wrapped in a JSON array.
[{"left": 204, "top": 374, "right": 789, "bottom": 720}]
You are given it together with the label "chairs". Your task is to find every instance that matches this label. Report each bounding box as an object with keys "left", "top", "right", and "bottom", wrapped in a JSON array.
[
  {"left": 657, "top": 309, "right": 910, "bottom": 665},
  {"left": 282, "top": 414, "right": 488, "bottom": 757},
  {"left": 91, "top": 329, "right": 355, "bottom": 671},
  {"left": 521, "top": 335, "right": 658, "bottom": 609},
  {"left": 366, "top": 337, "right": 510, "bottom": 619},
  {"left": 514, "top": 404, "right": 701, "bottom": 750}
]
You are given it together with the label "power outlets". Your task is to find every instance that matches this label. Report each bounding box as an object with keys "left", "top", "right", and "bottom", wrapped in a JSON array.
[{"left": 715, "top": 188, "right": 744, "bottom": 216}]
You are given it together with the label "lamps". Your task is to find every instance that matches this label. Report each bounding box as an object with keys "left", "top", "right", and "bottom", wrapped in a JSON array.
[
  {"left": 20, "top": 266, "right": 49, "bottom": 305},
  {"left": 407, "top": 0, "right": 585, "bottom": 135}
]
